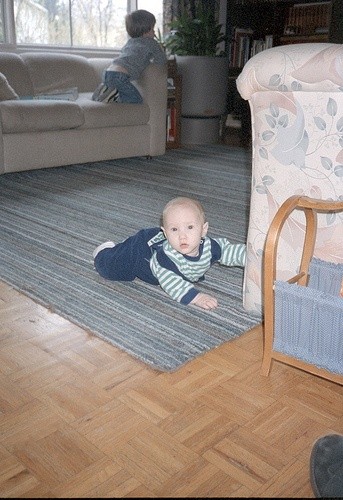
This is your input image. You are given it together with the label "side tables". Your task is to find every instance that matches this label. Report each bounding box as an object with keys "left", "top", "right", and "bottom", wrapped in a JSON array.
[{"left": 163, "top": 74, "right": 183, "bottom": 147}]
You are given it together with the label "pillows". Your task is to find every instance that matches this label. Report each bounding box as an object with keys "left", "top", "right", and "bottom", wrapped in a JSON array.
[{"left": 0, "top": 71, "right": 20, "bottom": 100}]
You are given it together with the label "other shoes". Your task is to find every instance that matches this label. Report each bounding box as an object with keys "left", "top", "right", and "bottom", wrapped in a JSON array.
[{"left": 92, "top": 241, "right": 115, "bottom": 259}]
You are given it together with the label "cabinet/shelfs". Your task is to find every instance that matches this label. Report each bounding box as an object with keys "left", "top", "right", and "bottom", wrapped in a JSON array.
[{"left": 225, "top": 0, "right": 343, "bottom": 128}]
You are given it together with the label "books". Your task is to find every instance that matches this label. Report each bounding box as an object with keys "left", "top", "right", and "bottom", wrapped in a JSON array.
[{"left": 228, "top": 3, "right": 333, "bottom": 69}]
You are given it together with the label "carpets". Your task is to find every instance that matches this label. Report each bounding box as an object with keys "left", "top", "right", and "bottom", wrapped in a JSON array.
[{"left": 0, "top": 137, "right": 266, "bottom": 372}]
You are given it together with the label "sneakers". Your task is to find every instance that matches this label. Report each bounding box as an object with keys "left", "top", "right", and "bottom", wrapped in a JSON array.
[{"left": 92, "top": 82, "right": 120, "bottom": 103}]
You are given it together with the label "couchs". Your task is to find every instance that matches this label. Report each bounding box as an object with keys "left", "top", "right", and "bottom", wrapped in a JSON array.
[
  {"left": 0, "top": 50, "right": 168, "bottom": 176},
  {"left": 233, "top": 44, "right": 343, "bottom": 316}
]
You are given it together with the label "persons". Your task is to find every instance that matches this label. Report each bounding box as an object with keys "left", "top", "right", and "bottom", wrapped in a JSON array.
[
  {"left": 92, "top": 196, "right": 248, "bottom": 310},
  {"left": 92, "top": 9, "right": 167, "bottom": 104}
]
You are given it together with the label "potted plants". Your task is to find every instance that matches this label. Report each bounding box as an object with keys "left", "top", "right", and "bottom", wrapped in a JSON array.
[{"left": 165, "top": 13, "right": 237, "bottom": 116}]
